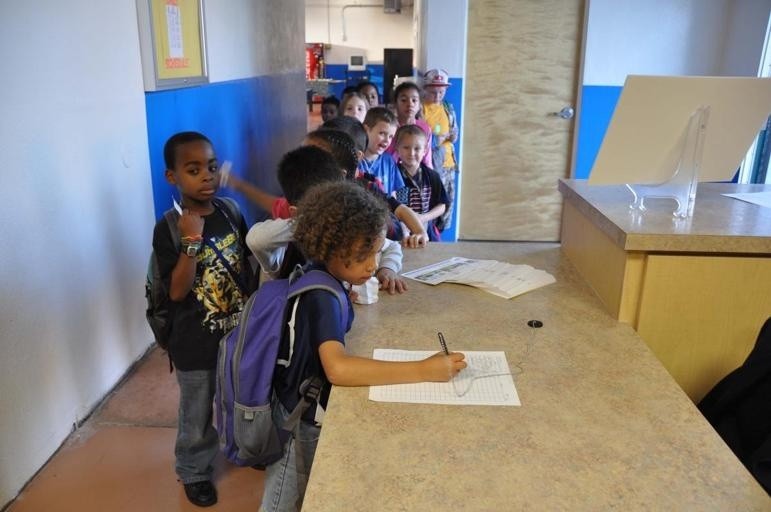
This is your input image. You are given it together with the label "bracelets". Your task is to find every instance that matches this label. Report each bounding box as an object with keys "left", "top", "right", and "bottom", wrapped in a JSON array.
[{"left": 182, "top": 233, "right": 202, "bottom": 241}]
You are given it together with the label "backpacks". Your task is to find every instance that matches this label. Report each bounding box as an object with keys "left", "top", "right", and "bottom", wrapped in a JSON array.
[
  {"left": 146, "top": 197, "right": 242, "bottom": 350},
  {"left": 212, "top": 264, "right": 348, "bottom": 466}
]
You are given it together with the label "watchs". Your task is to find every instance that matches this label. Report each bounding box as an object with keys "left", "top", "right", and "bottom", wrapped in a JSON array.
[{"left": 182, "top": 243, "right": 202, "bottom": 257}]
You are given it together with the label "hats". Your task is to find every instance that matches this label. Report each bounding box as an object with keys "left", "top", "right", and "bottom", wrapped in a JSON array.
[{"left": 424, "top": 68, "right": 452, "bottom": 86}]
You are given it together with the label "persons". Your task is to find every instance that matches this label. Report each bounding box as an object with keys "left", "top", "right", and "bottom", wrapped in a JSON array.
[
  {"left": 216, "top": 130, "right": 358, "bottom": 221},
  {"left": 314, "top": 66, "right": 459, "bottom": 248},
  {"left": 255, "top": 180, "right": 468, "bottom": 512},
  {"left": 145, "top": 129, "right": 259, "bottom": 508},
  {"left": 242, "top": 146, "right": 408, "bottom": 304}
]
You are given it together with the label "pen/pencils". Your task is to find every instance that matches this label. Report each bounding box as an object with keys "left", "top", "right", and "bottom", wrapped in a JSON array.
[{"left": 438, "top": 332, "right": 449, "bottom": 355}]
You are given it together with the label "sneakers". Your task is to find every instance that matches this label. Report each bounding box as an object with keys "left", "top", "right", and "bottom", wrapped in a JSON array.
[{"left": 184, "top": 480, "right": 217, "bottom": 506}]
